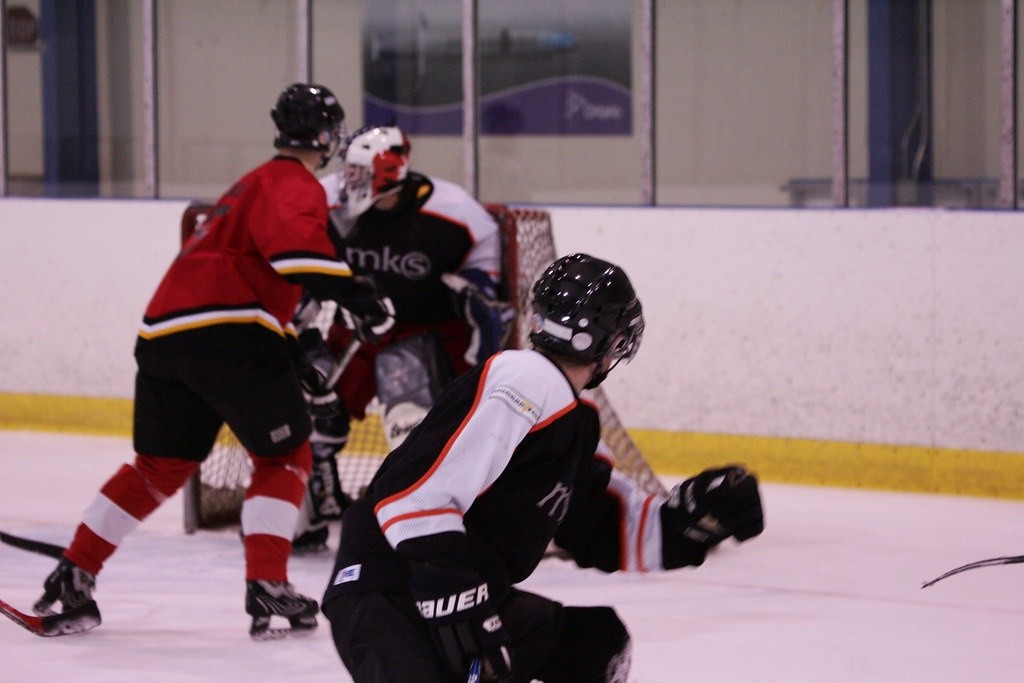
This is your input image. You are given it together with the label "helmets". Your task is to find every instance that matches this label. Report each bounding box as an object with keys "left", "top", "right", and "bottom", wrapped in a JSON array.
[
  {"left": 344, "top": 125, "right": 412, "bottom": 218},
  {"left": 272, "top": 81, "right": 343, "bottom": 150},
  {"left": 530, "top": 253, "right": 642, "bottom": 364}
]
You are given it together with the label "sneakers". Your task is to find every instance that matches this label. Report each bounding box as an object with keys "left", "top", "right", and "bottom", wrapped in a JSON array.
[
  {"left": 288, "top": 518, "right": 329, "bottom": 555},
  {"left": 35, "top": 555, "right": 95, "bottom": 616},
  {"left": 247, "top": 580, "right": 320, "bottom": 639}
]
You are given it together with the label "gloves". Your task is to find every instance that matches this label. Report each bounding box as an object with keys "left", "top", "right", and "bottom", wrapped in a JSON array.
[
  {"left": 412, "top": 573, "right": 510, "bottom": 682},
  {"left": 342, "top": 274, "right": 398, "bottom": 343},
  {"left": 670, "top": 464, "right": 764, "bottom": 550}
]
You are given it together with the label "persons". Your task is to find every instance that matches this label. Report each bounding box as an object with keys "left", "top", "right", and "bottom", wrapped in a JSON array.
[
  {"left": 320, "top": 253, "right": 766, "bottom": 683},
  {"left": 44, "top": 83, "right": 502, "bottom": 620}
]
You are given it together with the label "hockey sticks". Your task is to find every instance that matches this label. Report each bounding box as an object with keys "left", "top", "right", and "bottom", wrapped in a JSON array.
[
  {"left": 0, "top": 530, "right": 70, "bottom": 560},
  {"left": 0, "top": 597, "right": 105, "bottom": 641},
  {"left": 323, "top": 313, "right": 377, "bottom": 391},
  {"left": 466, "top": 657, "right": 483, "bottom": 683},
  {"left": 918, "top": 553, "right": 1023, "bottom": 591}
]
